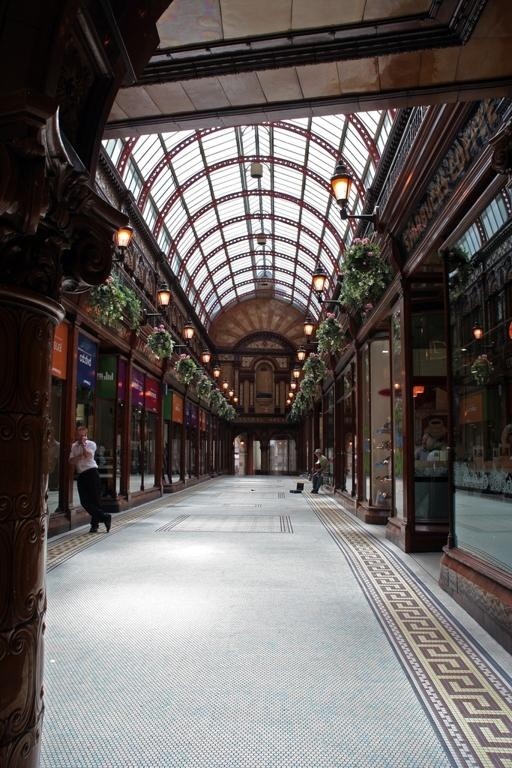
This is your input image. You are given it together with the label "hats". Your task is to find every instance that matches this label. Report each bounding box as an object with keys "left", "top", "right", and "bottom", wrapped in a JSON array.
[{"left": 312, "top": 449, "right": 324, "bottom": 454}]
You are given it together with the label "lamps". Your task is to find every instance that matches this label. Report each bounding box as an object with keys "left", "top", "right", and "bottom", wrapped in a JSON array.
[
  {"left": 251, "top": 158, "right": 263, "bottom": 177},
  {"left": 112, "top": 224, "right": 133, "bottom": 265},
  {"left": 257, "top": 233, "right": 266, "bottom": 245},
  {"left": 329, "top": 158, "right": 379, "bottom": 223}
]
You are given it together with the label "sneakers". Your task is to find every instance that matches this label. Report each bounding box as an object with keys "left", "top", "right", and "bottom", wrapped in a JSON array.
[
  {"left": 90, "top": 527, "right": 98, "bottom": 533},
  {"left": 311, "top": 489, "right": 318, "bottom": 494},
  {"left": 104, "top": 513, "right": 112, "bottom": 533}
]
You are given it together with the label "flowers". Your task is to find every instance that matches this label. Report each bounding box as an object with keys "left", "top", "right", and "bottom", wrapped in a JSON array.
[
  {"left": 284, "top": 237, "right": 391, "bottom": 426},
  {"left": 103, "top": 280, "right": 238, "bottom": 422}
]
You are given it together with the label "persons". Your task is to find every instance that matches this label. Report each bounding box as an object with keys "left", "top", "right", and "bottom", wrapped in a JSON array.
[
  {"left": 310, "top": 448, "right": 330, "bottom": 494},
  {"left": 415, "top": 432, "right": 448, "bottom": 519},
  {"left": 46, "top": 426, "right": 61, "bottom": 458},
  {"left": 69, "top": 423, "right": 112, "bottom": 533}
]
